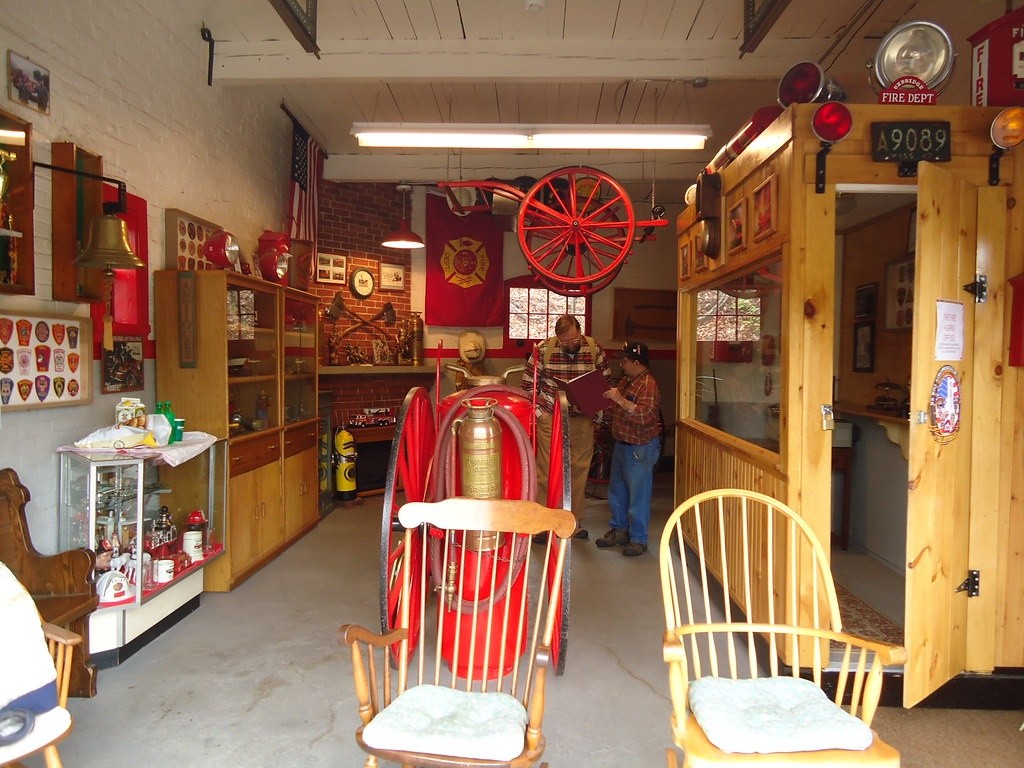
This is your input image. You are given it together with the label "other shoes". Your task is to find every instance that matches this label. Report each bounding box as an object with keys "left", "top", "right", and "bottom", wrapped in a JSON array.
[
  {"left": 556, "top": 529, "right": 588, "bottom": 538},
  {"left": 533, "top": 532, "right": 546, "bottom": 543}
]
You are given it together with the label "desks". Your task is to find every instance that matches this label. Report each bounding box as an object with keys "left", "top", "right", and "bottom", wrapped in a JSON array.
[{"left": 746, "top": 439, "right": 856, "bottom": 551}]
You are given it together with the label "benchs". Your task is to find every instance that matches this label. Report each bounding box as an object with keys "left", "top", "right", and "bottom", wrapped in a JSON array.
[{"left": 0, "top": 468, "right": 100, "bottom": 699}]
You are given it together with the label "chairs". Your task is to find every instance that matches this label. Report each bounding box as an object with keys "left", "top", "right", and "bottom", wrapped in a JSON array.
[
  {"left": 0, "top": 563, "right": 82, "bottom": 768},
  {"left": 662, "top": 487, "right": 906, "bottom": 768},
  {"left": 337, "top": 497, "right": 578, "bottom": 768}
]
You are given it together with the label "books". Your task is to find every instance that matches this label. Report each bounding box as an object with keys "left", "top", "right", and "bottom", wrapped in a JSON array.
[{"left": 553, "top": 369, "right": 616, "bottom": 417}]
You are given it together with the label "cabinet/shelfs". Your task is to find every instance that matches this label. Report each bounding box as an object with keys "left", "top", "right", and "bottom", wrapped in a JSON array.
[
  {"left": 59, "top": 437, "right": 228, "bottom": 670},
  {"left": 153, "top": 268, "right": 322, "bottom": 593}
]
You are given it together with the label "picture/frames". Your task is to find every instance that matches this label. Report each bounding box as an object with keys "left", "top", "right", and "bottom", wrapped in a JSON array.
[
  {"left": 852, "top": 282, "right": 876, "bottom": 372},
  {"left": 316, "top": 251, "right": 406, "bottom": 298},
  {"left": 884, "top": 252, "right": 913, "bottom": 332},
  {"left": 7, "top": 50, "right": 51, "bottom": 116}
]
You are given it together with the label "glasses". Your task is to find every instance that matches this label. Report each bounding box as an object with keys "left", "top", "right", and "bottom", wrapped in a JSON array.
[{"left": 559, "top": 330, "right": 580, "bottom": 345}]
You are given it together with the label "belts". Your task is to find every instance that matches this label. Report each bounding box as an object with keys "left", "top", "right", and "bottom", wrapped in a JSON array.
[{"left": 617, "top": 440, "right": 634, "bottom": 446}]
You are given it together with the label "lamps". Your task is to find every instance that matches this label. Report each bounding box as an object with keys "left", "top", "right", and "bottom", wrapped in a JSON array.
[
  {"left": 811, "top": 102, "right": 852, "bottom": 194},
  {"left": 348, "top": 80, "right": 713, "bottom": 149},
  {"left": 202, "top": 229, "right": 288, "bottom": 283},
  {"left": 989, "top": 107, "right": 1024, "bottom": 187},
  {"left": 778, "top": 62, "right": 848, "bottom": 103},
  {"left": 381, "top": 184, "right": 426, "bottom": 249},
  {"left": 865, "top": 19, "right": 959, "bottom": 97}
]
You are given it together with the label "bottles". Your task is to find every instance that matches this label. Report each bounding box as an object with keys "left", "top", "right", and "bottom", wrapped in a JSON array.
[
  {"left": 154, "top": 402, "right": 164, "bottom": 414},
  {"left": 154, "top": 505, "right": 177, "bottom": 542},
  {"left": 164, "top": 401, "right": 176, "bottom": 444}
]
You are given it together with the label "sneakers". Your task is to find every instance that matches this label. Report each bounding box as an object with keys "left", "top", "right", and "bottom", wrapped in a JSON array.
[
  {"left": 623, "top": 541, "right": 647, "bottom": 557},
  {"left": 595, "top": 528, "right": 629, "bottom": 547}
]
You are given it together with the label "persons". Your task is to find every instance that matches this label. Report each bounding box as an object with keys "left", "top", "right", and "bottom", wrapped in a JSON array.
[
  {"left": 13, "top": 73, "right": 49, "bottom": 112},
  {"left": 595, "top": 341, "right": 661, "bottom": 557},
  {"left": 520, "top": 314, "right": 613, "bottom": 542}
]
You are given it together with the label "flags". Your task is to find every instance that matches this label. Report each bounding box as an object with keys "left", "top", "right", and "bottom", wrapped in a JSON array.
[
  {"left": 425, "top": 192, "right": 505, "bottom": 326},
  {"left": 293, "top": 123, "right": 319, "bottom": 242}
]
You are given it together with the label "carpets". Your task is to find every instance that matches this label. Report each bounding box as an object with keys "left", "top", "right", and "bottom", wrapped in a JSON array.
[{"left": 831, "top": 581, "right": 905, "bottom": 652}]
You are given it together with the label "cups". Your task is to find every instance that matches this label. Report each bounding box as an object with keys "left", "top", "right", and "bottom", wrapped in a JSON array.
[
  {"left": 141, "top": 557, "right": 159, "bottom": 591},
  {"left": 183, "top": 531, "right": 203, "bottom": 556},
  {"left": 174, "top": 418, "right": 185, "bottom": 441},
  {"left": 158, "top": 560, "right": 174, "bottom": 583}
]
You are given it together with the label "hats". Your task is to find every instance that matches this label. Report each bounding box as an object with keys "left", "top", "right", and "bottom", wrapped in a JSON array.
[{"left": 615, "top": 342, "right": 649, "bottom": 365}]
